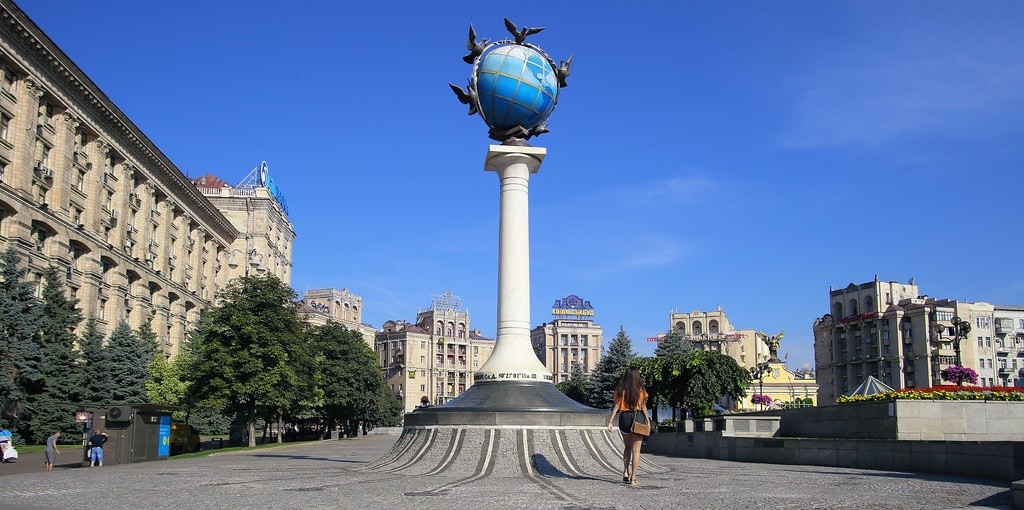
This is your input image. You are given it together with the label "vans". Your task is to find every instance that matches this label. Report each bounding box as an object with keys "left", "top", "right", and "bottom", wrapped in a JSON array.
[{"left": 169, "top": 419, "right": 201, "bottom": 453}]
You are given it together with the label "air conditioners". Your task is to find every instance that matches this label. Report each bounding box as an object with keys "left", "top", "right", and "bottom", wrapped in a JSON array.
[{"left": 105, "top": 406, "right": 134, "bottom": 423}]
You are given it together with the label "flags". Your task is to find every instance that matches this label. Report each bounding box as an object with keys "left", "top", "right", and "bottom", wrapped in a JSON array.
[{"left": 785, "top": 351, "right": 788, "bottom": 359}]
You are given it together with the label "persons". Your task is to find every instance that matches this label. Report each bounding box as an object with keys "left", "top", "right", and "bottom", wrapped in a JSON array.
[
  {"left": 88, "top": 429, "right": 109, "bottom": 467},
  {"left": 608, "top": 366, "right": 650, "bottom": 487},
  {"left": 0, "top": 424, "right": 12, "bottom": 463},
  {"left": 415, "top": 397, "right": 431, "bottom": 409},
  {"left": 768, "top": 335, "right": 781, "bottom": 358},
  {"left": 642, "top": 403, "right": 690, "bottom": 445}
]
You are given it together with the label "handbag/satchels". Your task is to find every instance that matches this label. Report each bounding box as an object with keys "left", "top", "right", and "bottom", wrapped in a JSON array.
[{"left": 630, "top": 421, "right": 651, "bottom": 437}]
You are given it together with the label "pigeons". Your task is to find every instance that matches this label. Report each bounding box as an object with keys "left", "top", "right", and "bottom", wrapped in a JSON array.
[
  {"left": 549, "top": 53, "right": 574, "bottom": 88},
  {"left": 504, "top": 17, "right": 547, "bottom": 45},
  {"left": 448, "top": 77, "right": 479, "bottom": 116},
  {"left": 463, "top": 23, "right": 492, "bottom": 65}
]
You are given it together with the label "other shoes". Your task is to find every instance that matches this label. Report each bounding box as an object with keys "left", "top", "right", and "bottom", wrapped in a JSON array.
[
  {"left": 631, "top": 480, "right": 638, "bottom": 486},
  {"left": 623, "top": 473, "right": 629, "bottom": 482}
]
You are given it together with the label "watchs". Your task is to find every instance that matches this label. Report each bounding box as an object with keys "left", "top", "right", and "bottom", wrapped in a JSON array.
[{"left": 45, "top": 432, "right": 61, "bottom": 472}]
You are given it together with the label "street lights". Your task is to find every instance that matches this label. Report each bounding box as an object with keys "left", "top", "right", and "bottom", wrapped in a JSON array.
[
  {"left": 750, "top": 362, "right": 773, "bottom": 411},
  {"left": 229, "top": 248, "right": 266, "bottom": 288},
  {"left": 934, "top": 316, "right": 972, "bottom": 386}
]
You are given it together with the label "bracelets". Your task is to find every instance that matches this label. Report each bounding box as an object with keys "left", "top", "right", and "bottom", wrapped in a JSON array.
[{"left": 609, "top": 422, "right": 613, "bottom": 424}]
